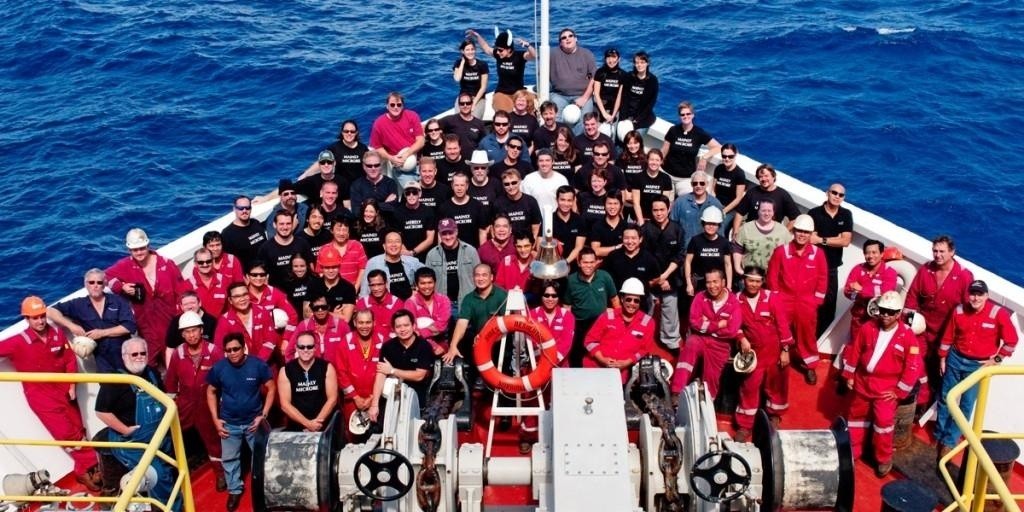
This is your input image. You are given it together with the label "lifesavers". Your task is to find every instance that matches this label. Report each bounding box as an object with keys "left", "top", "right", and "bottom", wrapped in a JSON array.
[
  {"left": 472, "top": 314, "right": 558, "bottom": 393},
  {"left": 885, "top": 259, "right": 917, "bottom": 304}
]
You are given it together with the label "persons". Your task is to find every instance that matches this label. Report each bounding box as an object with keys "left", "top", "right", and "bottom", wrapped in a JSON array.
[
  {"left": 452, "top": 39, "right": 489, "bottom": 120},
  {"left": 464, "top": 29, "right": 536, "bottom": 115},
  {"left": 593, "top": 48, "right": 627, "bottom": 145},
  {"left": 619, "top": 51, "right": 658, "bottom": 151},
  {"left": 550, "top": 28, "right": 597, "bottom": 137}
]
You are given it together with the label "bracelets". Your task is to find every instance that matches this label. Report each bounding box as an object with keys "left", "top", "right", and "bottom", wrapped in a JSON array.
[{"left": 526, "top": 44, "right": 530, "bottom": 48}]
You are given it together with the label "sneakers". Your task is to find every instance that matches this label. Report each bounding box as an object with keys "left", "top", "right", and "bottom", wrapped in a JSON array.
[
  {"left": 807, "top": 369, "right": 817, "bottom": 385},
  {"left": 226, "top": 494, "right": 239, "bottom": 510},
  {"left": 877, "top": 462, "right": 892, "bottom": 478},
  {"left": 216, "top": 477, "right": 227, "bottom": 490},
  {"left": 520, "top": 440, "right": 531, "bottom": 454},
  {"left": 940, "top": 446, "right": 956, "bottom": 462},
  {"left": 735, "top": 430, "right": 749, "bottom": 442}
]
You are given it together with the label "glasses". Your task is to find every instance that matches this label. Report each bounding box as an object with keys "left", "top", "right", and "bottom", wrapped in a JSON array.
[
  {"left": 312, "top": 304, "right": 328, "bottom": 312},
  {"left": 132, "top": 352, "right": 146, "bottom": 356},
  {"left": 319, "top": 102, "right": 523, "bottom": 195},
  {"left": 561, "top": 35, "right": 573, "bottom": 39},
  {"left": 87, "top": 281, "right": 104, "bottom": 285},
  {"left": 722, "top": 154, "right": 735, "bottom": 158},
  {"left": 543, "top": 294, "right": 557, "bottom": 298},
  {"left": 593, "top": 151, "right": 609, "bottom": 156},
  {"left": 829, "top": 190, "right": 845, "bottom": 198},
  {"left": 681, "top": 112, "right": 692, "bottom": 116},
  {"left": 284, "top": 192, "right": 296, "bottom": 196},
  {"left": 625, "top": 298, "right": 640, "bottom": 304},
  {"left": 297, "top": 344, "right": 314, "bottom": 349},
  {"left": 691, "top": 182, "right": 706, "bottom": 186},
  {"left": 235, "top": 205, "right": 251, "bottom": 210},
  {"left": 249, "top": 273, "right": 267, "bottom": 277},
  {"left": 225, "top": 347, "right": 241, "bottom": 353},
  {"left": 197, "top": 260, "right": 212, "bottom": 264},
  {"left": 497, "top": 48, "right": 505, "bottom": 53}
]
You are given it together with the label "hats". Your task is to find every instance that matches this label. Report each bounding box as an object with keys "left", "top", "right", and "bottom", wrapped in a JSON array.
[
  {"left": 494, "top": 26, "right": 514, "bottom": 49},
  {"left": 438, "top": 218, "right": 457, "bottom": 233},
  {"left": 969, "top": 280, "right": 988, "bottom": 294},
  {"left": 279, "top": 179, "right": 294, "bottom": 194}
]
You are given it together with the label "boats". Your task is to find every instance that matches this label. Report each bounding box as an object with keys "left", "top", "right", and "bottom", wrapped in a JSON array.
[{"left": 0, "top": 0, "right": 1024, "bottom": 512}]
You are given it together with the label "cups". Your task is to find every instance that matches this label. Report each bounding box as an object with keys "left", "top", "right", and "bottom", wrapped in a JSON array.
[{"left": 131, "top": 282, "right": 144, "bottom": 304}]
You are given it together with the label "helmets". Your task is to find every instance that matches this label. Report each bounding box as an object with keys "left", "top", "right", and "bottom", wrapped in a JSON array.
[
  {"left": 733, "top": 348, "right": 757, "bottom": 373},
  {"left": 126, "top": 228, "right": 149, "bottom": 249},
  {"left": 178, "top": 311, "right": 204, "bottom": 329},
  {"left": 793, "top": 214, "right": 814, "bottom": 232},
  {"left": 619, "top": 277, "right": 647, "bottom": 295},
  {"left": 599, "top": 122, "right": 614, "bottom": 138},
  {"left": 348, "top": 409, "right": 370, "bottom": 436},
  {"left": 272, "top": 308, "right": 288, "bottom": 330},
  {"left": 700, "top": 205, "right": 724, "bottom": 224},
  {"left": 867, "top": 291, "right": 927, "bottom": 334},
  {"left": 319, "top": 247, "right": 342, "bottom": 267},
  {"left": 617, "top": 119, "right": 634, "bottom": 143},
  {"left": 562, "top": 104, "right": 581, "bottom": 124},
  {"left": 417, "top": 316, "right": 434, "bottom": 329},
  {"left": 21, "top": 296, "right": 47, "bottom": 316},
  {"left": 72, "top": 336, "right": 97, "bottom": 358}
]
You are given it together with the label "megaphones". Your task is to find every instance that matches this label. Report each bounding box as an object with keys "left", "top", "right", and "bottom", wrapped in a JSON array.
[
  {"left": 3, "top": 469, "right": 50, "bottom": 507},
  {"left": 120, "top": 464, "right": 158, "bottom": 495}
]
